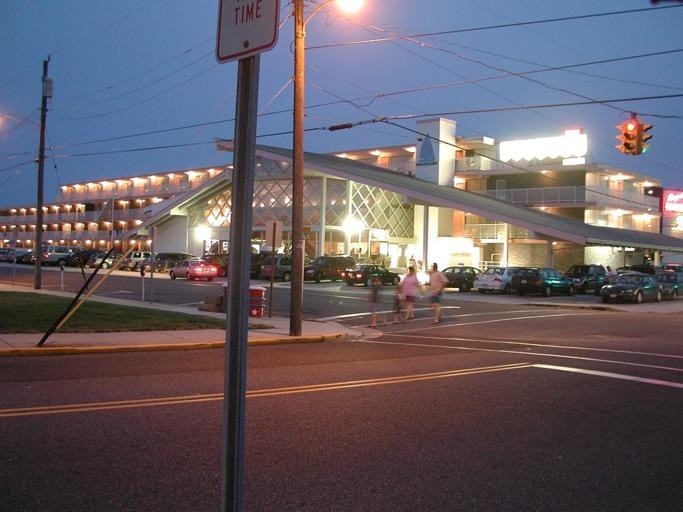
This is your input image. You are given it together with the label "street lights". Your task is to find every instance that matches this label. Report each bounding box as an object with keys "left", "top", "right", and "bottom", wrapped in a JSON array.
[{"left": 289, "top": 1, "right": 367, "bottom": 336}]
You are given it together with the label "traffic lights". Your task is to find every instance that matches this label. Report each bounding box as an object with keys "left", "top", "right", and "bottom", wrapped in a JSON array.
[{"left": 613, "top": 114, "right": 652, "bottom": 154}]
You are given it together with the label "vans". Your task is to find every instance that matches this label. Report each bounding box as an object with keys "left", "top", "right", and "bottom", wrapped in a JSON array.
[{"left": 567, "top": 264, "right": 606, "bottom": 294}]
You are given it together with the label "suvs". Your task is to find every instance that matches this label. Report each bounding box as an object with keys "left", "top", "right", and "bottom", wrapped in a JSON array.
[
  {"left": 221, "top": 255, "right": 269, "bottom": 278},
  {"left": 304, "top": 255, "right": 357, "bottom": 283}
]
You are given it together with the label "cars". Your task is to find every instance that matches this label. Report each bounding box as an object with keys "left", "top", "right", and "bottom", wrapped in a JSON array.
[
  {"left": 474, "top": 266, "right": 533, "bottom": 294},
  {"left": 600, "top": 262, "right": 683, "bottom": 304},
  {"left": 442, "top": 265, "right": 481, "bottom": 291},
  {"left": 261, "top": 253, "right": 314, "bottom": 280},
  {"left": 512, "top": 267, "right": 575, "bottom": 296},
  {"left": 0, "top": 245, "right": 199, "bottom": 273},
  {"left": 169, "top": 257, "right": 217, "bottom": 281},
  {"left": 342, "top": 261, "right": 400, "bottom": 286}
]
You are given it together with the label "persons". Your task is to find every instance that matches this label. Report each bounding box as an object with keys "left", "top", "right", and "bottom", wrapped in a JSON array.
[
  {"left": 393, "top": 287, "right": 403, "bottom": 323},
  {"left": 429, "top": 263, "right": 450, "bottom": 323},
  {"left": 403, "top": 266, "right": 422, "bottom": 321},
  {"left": 367, "top": 279, "right": 380, "bottom": 327}
]
